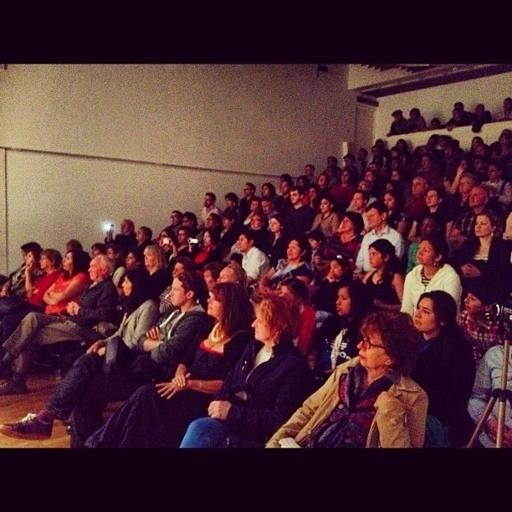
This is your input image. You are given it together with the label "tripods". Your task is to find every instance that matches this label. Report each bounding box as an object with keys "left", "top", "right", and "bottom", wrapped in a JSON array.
[{"left": 463, "top": 336, "right": 512, "bottom": 448}]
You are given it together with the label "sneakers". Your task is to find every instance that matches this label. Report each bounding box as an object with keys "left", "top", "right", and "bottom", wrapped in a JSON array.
[{"left": 0, "top": 413, "right": 53, "bottom": 440}]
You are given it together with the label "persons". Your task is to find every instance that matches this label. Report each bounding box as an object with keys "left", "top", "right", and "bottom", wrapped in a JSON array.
[
  {"left": 446, "top": 108, "right": 472, "bottom": 132},
  {"left": 385, "top": 110, "right": 408, "bottom": 137},
  {"left": 428, "top": 117, "right": 442, "bottom": 129},
  {"left": 1, "top": 127, "right": 511, "bottom": 448},
  {"left": 470, "top": 103, "right": 494, "bottom": 133},
  {"left": 408, "top": 108, "right": 427, "bottom": 131},
  {"left": 497, "top": 97, "right": 512, "bottom": 120},
  {"left": 449, "top": 102, "right": 474, "bottom": 121}
]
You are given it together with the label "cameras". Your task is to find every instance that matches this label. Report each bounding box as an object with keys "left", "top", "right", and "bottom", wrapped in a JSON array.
[
  {"left": 489, "top": 301, "right": 512, "bottom": 336},
  {"left": 188, "top": 237, "right": 199, "bottom": 244},
  {"left": 162, "top": 238, "right": 169, "bottom": 244},
  {"left": 105, "top": 223, "right": 114, "bottom": 235}
]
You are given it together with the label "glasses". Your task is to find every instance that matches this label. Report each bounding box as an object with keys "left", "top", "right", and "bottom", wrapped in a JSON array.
[{"left": 361, "top": 339, "right": 385, "bottom": 349}]
